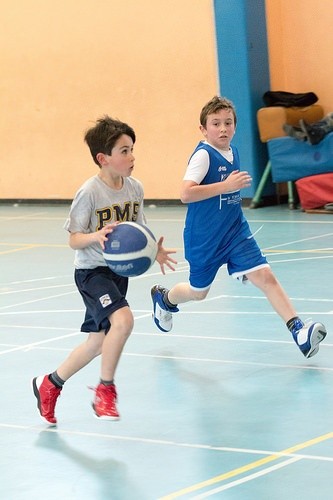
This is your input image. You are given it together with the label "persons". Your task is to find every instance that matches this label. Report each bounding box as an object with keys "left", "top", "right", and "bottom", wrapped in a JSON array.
[
  {"left": 32, "top": 115, "right": 177, "bottom": 425},
  {"left": 152, "top": 95, "right": 326, "bottom": 357}
]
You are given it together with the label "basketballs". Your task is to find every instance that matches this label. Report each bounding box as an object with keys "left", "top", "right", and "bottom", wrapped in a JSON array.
[{"left": 102, "top": 222, "right": 158, "bottom": 277}]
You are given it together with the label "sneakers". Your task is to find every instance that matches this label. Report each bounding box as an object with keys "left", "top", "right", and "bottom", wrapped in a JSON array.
[
  {"left": 89, "top": 382, "right": 120, "bottom": 419},
  {"left": 292, "top": 319, "right": 327, "bottom": 358},
  {"left": 151, "top": 285, "right": 179, "bottom": 332},
  {"left": 33, "top": 373, "right": 63, "bottom": 427}
]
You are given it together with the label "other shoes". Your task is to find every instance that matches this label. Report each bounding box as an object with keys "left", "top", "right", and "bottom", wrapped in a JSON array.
[
  {"left": 282, "top": 123, "right": 308, "bottom": 142},
  {"left": 299, "top": 119, "right": 323, "bottom": 145}
]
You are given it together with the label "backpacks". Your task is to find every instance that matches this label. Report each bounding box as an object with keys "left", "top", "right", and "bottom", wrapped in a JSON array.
[{"left": 262, "top": 91, "right": 318, "bottom": 108}]
